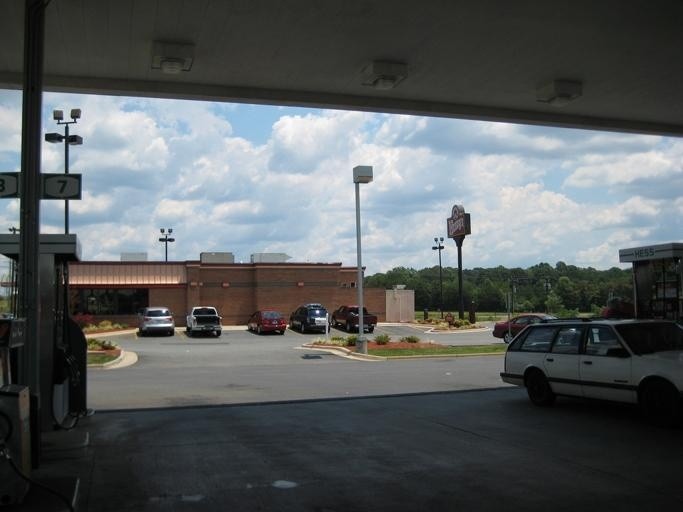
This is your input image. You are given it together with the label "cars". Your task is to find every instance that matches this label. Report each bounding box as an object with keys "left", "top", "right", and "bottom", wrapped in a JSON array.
[
  {"left": 498, "top": 315, "right": 682, "bottom": 424},
  {"left": 245, "top": 308, "right": 288, "bottom": 336},
  {"left": 491, "top": 311, "right": 560, "bottom": 346},
  {"left": 1, "top": 313, "right": 14, "bottom": 320}
]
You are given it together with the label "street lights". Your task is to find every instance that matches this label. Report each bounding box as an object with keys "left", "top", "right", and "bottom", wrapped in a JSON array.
[
  {"left": 508, "top": 276, "right": 517, "bottom": 314},
  {"left": 431, "top": 235, "right": 446, "bottom": 319},
  {"left": 44, "top": 106, "right": 81, "bottom": 321},
  {"left": 157, "top": 228, "right": 175, "bottom": 261},
  {"left": 352, "top": 164, "right": 375, "bottom": 358},
  {"left": 4, "top": 226, "right": 20, "bottom": 313}
]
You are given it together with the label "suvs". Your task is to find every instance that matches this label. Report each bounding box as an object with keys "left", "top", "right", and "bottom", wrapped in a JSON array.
[
  {"left": 289, "top": 302, "right": 332, "bottom": 336},
  {"left": 136, "top": 305, "right": 175, "bottom": 336}
]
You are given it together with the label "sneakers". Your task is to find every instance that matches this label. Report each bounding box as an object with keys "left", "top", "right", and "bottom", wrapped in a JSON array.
[{"left": 70, "top": 408, "right": 95, "bottom": 418}]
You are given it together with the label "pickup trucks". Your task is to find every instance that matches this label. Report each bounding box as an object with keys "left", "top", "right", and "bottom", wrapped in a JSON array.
[
  {"left": 331, "top": 304, "right": 378, "bottom": 335},
  {"left": 184, "top": 305, "right": 224, "bottom": 339}
]
